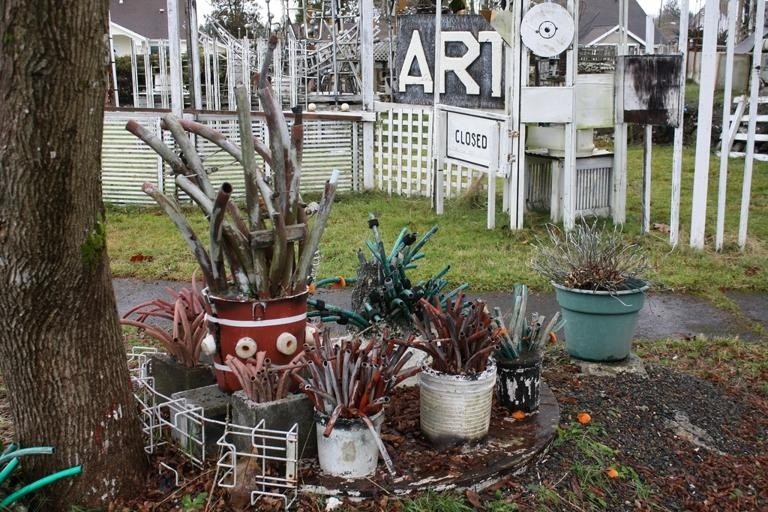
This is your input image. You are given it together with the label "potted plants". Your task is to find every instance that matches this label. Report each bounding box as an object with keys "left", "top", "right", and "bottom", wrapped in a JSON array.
[{"left": 536, "top": 220, "right": 669, "bottom": 362}]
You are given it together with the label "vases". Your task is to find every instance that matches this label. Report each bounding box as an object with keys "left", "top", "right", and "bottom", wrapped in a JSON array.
[
  {"left": 311, "top": 405, "right": 384, "bottom": 477},
  {"left": 418, "top": 354, "right": 497, "bottom": 444},
  {"left": 201, "top": 284, "right": 309, "bottom": 391}
]
